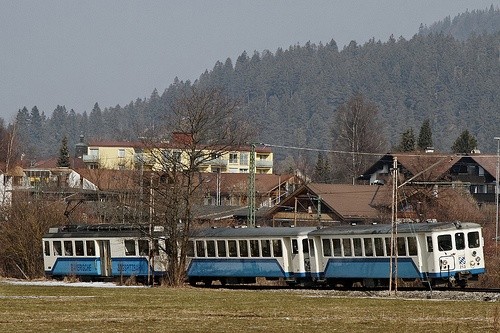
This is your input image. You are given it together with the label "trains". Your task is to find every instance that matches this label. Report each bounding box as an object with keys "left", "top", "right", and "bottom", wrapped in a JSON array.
[{"left": 42, "top": 220, "right": 485, "bottom": 288}]
